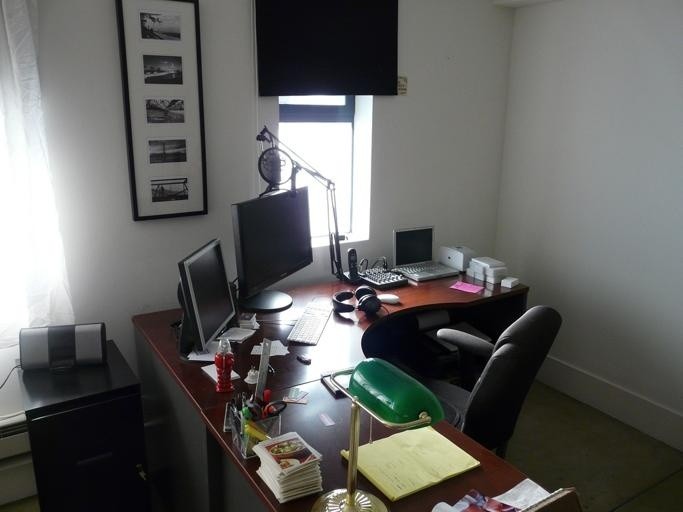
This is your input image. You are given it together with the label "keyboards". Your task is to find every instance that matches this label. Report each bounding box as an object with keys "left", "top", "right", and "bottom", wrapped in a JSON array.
[{"left": 287, "top": 305, "right": 333, "bottom": 346}]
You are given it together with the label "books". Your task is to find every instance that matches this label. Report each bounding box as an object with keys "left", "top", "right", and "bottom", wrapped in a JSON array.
[
  {"left": 340, "top": 426, "right": 481, "bottom": 501},
  {"left": 252, "top": 432, "right": 324, "bottom": 504}
]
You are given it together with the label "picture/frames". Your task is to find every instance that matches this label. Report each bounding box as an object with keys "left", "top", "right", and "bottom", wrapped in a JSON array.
[{"left": 116, "top": 0, "right": 208, "bottom": 220}]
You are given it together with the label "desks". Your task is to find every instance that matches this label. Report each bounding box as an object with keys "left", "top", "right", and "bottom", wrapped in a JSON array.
[{"left": 131, "top": 270, "right": 551, "bottom": 512}]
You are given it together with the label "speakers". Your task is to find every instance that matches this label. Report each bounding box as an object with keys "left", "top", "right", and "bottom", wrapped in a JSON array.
[{"left": 19, "top": 323, "right": 107, "bottom": 369}]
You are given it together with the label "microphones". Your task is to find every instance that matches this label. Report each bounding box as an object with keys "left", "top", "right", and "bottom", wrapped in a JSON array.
[{"left": 256, "top": 135, "right": 272, "bottom": 144}]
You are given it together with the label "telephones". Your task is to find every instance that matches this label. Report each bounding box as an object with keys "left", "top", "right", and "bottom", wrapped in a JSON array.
[{"left": 343, "top": 248, "right": 361, "bottom": 285}]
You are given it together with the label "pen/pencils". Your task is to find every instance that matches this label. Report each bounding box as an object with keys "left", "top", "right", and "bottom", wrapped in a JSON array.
[{"left": 229, "top": 390, "right": 275, "bottom": 453}]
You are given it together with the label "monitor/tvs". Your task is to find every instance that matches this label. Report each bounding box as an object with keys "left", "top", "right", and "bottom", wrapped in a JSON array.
[
  {"left": 176, "top": 236, "right": 237, "bottom": 356},
  {"left": 232, "top": 186, "right": 313, "bottom": 313}
]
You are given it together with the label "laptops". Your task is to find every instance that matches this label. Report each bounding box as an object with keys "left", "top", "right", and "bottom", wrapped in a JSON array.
[{"left": 392, "top": 226, "right": 459, "bottom": 281}]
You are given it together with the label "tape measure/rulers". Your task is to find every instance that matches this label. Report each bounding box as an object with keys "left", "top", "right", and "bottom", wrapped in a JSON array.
[{"left": 255, "top": 338, "right": 271, "bottom": 398}]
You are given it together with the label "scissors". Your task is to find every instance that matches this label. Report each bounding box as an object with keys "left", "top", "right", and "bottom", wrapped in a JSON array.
[{"left": 248, "top": 400, "right": 287, "bottom": 434}]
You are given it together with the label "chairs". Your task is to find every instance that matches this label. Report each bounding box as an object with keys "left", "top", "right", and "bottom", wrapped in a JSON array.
[{"left": 426, "top": 305, "right": 562, "bottom": 461}]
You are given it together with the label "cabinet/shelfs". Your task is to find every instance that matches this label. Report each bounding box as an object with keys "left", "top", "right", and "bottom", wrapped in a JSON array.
[{"left": 0, "top": 339, "right": 145, "bottom": 512}]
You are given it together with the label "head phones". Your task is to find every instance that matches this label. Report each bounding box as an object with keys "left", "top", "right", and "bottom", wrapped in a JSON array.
[{"left": 331, "top": 286, "right": 381, "bottom": 313}]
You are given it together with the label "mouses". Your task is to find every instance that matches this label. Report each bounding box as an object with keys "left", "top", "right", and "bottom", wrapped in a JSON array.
[{"left": 377, "top": 293, "right": 400, "bottom": 305}]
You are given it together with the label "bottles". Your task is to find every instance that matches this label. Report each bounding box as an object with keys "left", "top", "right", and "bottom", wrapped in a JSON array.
[{"left": 214, "top": 338, "right": 234, "bottom": 394}]
